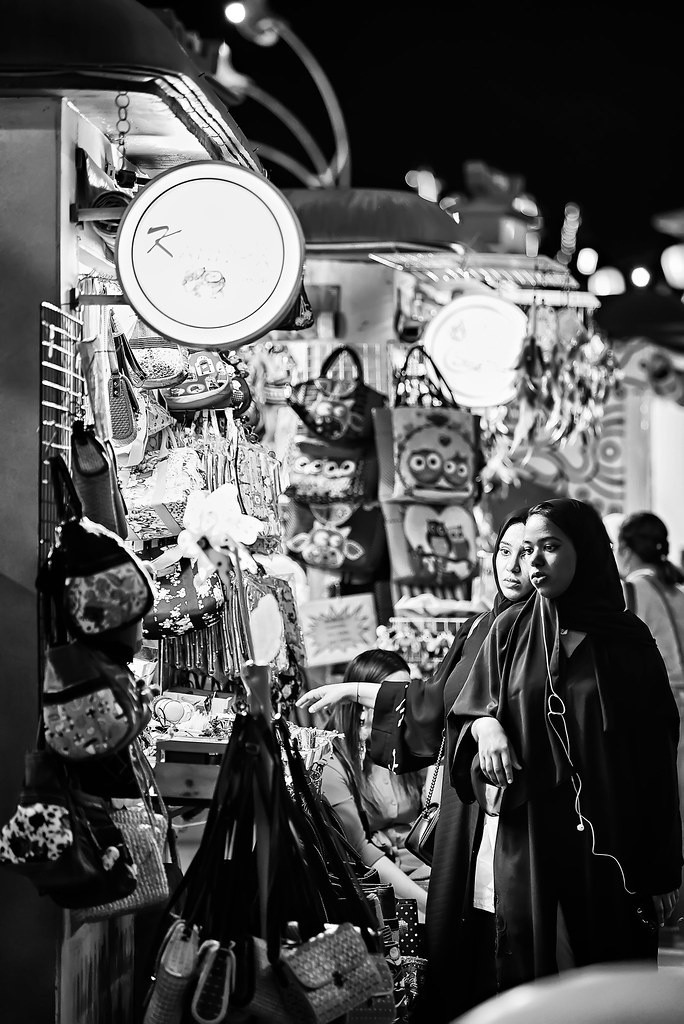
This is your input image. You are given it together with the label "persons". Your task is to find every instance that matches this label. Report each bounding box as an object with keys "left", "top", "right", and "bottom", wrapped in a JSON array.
[
  {"left": 293, "top": 507, "right": 535, "bottom": 1023},
  {"left": 612, "top": 508, "right": 684, "bottom": 843},
  {"left": 320, "top": 649, "right": 439, "bottom": 936},
  {"left": 445, "top": 497, "right": 684, "bottom": 997}
]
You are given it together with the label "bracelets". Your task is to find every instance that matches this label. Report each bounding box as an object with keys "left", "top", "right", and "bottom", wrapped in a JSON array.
[
  {"left": 356, "top": 682, "right": 361, "bottom": 703},
  {"left": 149, "top": 695, "right": 185, "bottom": 725}
]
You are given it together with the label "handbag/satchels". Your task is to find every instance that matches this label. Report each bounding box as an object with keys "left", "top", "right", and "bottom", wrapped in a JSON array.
[
  {"left": 282, "top": 345, "right": 483, "bottom": 586},
  {"left": 143, "top": 528, "right": 224, "bottom": 638},
  {"left": 404, "top": 802, "right": 441, "bottom": 869},
  {"left": 106, "top": 310, "right": 147, "bottom": 439},
  {"left": 0, "top": 430, "right": 171, "bottom": 927},
  {"left": 159, "top": 351, "right": 251, "bottom": 416},
  {"left": 139, "top": 713, "right": 397, "bottom": 1024}
]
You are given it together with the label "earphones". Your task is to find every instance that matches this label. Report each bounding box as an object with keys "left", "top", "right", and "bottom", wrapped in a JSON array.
[{"left": 577, "top": 816, "right": 584, "bottom": 831}]
